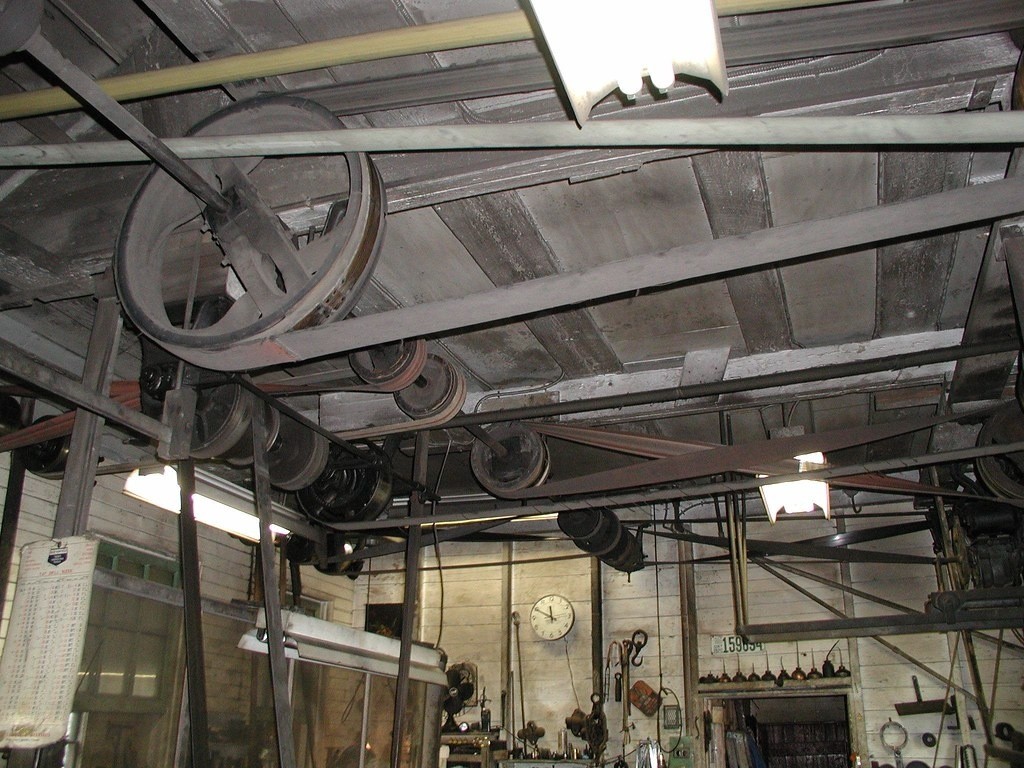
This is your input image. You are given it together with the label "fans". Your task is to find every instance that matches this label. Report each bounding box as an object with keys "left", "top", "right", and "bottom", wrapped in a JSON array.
[{"left": 442, "top": 670, "right": 477, "bottom": 734}]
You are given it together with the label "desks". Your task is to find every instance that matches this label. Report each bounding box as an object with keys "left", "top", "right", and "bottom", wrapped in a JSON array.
[{"left": 498, "top": 759, "right": 593, "bottom": 768}]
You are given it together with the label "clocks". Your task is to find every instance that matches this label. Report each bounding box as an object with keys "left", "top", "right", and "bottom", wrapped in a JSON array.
[{"left": 529, "top": 594, "right": 575, "bottom": 641}]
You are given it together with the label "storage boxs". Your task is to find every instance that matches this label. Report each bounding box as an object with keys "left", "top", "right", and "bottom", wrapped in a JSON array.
[{"left": 3, "top": 715, "right": 67, "bottom": 740}]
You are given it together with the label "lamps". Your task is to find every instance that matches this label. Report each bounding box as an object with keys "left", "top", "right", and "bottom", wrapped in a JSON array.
[
  {"left": 756, "top": 451, "right": 831, "bottom": 527},
  {"left": 121, "top": 465, "right": 306, "bottom": 548},
  {"left": 236, "top": 607, "right": 449, "bottom": 688}
]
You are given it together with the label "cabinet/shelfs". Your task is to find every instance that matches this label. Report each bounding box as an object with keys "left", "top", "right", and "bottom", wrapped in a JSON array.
[{"left": 441, "top": 732, "right": 498, "bottom": 768}]
